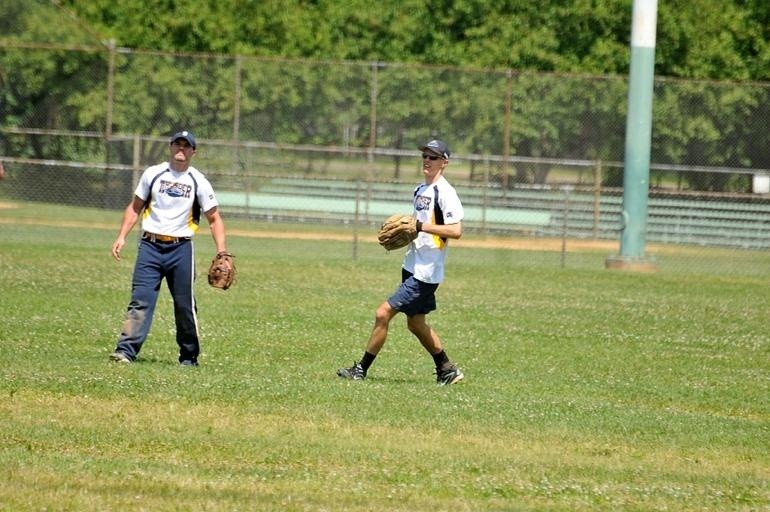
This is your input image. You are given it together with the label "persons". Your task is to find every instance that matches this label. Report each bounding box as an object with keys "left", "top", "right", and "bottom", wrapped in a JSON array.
[
  {"left": 110, "top": 131, "right": 236, "bottom": 367},
  {"left": 336, "top": 139, "right": 464, "bottom": 386}
]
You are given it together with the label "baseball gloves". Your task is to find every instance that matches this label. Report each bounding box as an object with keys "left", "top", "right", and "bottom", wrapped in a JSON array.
[
  {"left": 208, "top": 252, "right": 237, "bottom": 289},
  {"left": 379, "top": 213, "right": 418, "bottom": 250}
]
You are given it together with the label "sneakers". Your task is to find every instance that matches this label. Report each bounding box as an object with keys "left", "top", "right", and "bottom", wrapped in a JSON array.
[
  {"left": 179, "top": 359, "right": 199, "bottom": 367},
  {"left": 335, "top": 359, "right": 367, "bottom": 383},
  {"left": 431, "top": 362, "right": 465, "bottom": 387},
  {"left": 108, "top": 351, "right": 132, "bottom": 365}
]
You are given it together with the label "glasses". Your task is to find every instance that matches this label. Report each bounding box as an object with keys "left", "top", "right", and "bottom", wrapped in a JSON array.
[{"left": 421, "top": 153, "right": 445, "bottom": 163}]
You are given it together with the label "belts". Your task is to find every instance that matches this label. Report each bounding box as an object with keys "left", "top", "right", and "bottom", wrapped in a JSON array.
[{"left": 143, "top": 232, "right": 180, "bottom": 242}]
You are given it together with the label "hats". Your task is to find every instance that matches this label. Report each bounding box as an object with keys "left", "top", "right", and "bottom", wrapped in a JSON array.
[
  {"left": 416, "top": 139, "right": 451, "bottom": 162},
  {"left": 170, "top": 130, "right": 197, "bottom": 148}
]
